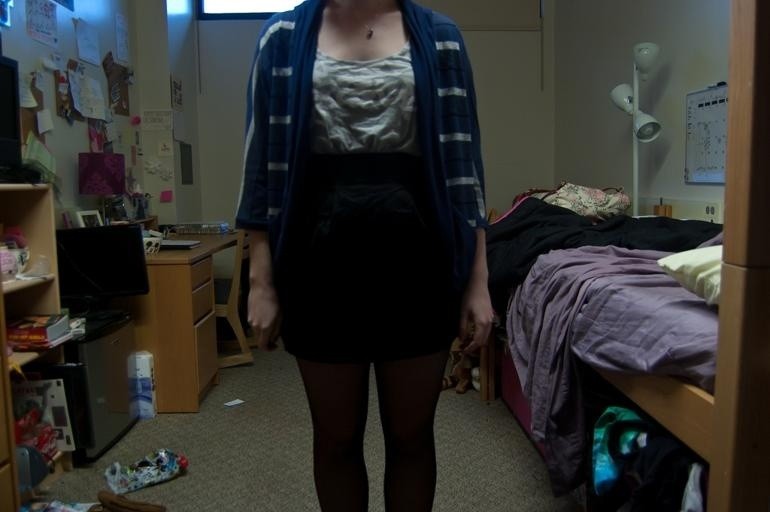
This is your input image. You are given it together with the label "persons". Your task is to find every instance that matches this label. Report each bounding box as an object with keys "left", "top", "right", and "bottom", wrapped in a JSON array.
[{"left": 232, "top": 1, "right": 496, "bottom": 512}]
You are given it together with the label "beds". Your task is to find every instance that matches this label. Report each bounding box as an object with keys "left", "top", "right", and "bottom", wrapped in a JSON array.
[{"left": 480, "top": 2, "right": 769, "bottom": 511}]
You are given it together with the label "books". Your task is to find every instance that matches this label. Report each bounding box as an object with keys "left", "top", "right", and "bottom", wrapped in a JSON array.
[
  {"left": 6, "top": 311, "right": 73, "bottom": 351},
  {"left": 159, "top": 238, "right": 204, "bottom": 249}
]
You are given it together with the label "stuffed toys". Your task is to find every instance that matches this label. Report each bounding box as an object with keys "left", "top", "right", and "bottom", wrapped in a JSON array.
[{"left": 441, "top": 340, "right": 481, "bottom": 395}]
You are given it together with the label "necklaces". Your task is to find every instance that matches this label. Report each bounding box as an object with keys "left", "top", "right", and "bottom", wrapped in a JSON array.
[{"left": 350, "top": 0, "right": 385, "bottom": 42}]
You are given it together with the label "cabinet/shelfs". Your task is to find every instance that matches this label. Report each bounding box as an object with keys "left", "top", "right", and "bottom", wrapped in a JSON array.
[{"left": 2, "top": 183, "right": 73, "bottom": 511}]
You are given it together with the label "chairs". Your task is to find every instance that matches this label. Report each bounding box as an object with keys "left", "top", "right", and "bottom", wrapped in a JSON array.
[{"left": 215, "top": 228, "right": 259, "bottom": 368}]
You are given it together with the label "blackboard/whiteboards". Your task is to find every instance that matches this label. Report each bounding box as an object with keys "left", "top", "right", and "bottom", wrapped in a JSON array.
[{"left": 684, "top": 84, "right": 729, "bottom": 186}]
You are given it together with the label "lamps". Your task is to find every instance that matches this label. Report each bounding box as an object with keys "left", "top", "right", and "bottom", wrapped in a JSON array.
[
  {"left": 77, "top": 151, "right": 125, "bottom": 224},
  {"left": 609, "top": 44, "right": 663, "bottom": 213}
]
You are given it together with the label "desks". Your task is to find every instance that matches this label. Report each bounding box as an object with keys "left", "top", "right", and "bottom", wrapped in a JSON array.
[{"left": 120, "top": 227, "right": 247, "bottom": 414}]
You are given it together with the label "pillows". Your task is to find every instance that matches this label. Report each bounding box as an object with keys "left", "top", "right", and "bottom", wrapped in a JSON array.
[{"left": 657, "top": 243, "right": 723, "bottom": 307}]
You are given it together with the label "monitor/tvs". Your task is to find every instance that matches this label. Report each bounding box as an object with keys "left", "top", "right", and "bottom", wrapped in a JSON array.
[{"left": 56, "top": 222, "right": 149, "bottom": 321}]
